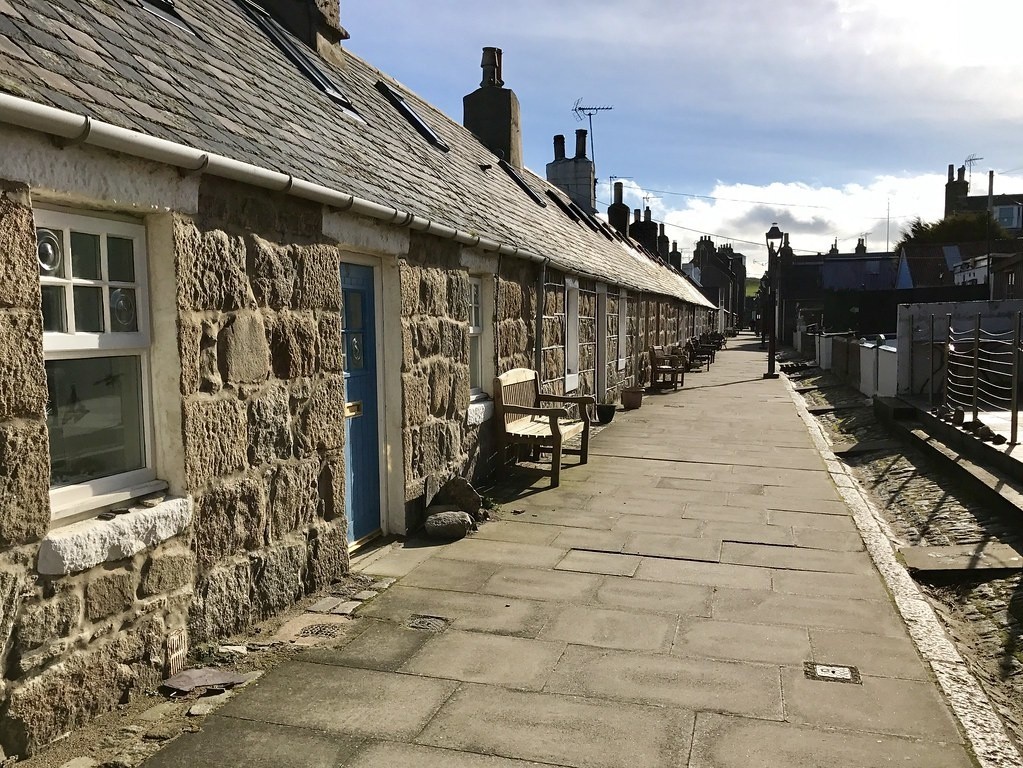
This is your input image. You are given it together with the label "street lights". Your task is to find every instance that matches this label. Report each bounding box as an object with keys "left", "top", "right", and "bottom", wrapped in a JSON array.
[
  {"left": 763, "top": 221, "right": 785, "bottom": 378},
  {"left": 754, "top": 275, "right": 768, "bottom": 344}
]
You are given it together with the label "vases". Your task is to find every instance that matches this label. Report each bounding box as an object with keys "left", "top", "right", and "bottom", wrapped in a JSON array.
[{"left": 622, "top": 386, "right": 645, "bottom": 409}]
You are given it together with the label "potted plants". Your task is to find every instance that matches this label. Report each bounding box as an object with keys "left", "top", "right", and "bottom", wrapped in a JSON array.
[{"left": 594, "top": 392, "right": 619, "bottom": 424}]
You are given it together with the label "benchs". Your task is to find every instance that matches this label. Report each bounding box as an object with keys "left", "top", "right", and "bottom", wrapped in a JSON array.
[
  {"left": 687, "top": 331, "right": 726, "bottom": 371},
  {"left": 650, "top": 345, "right": 684, "bottom": 391},
  {"left": 494, "top": 368, "right": 594, "bottom": 489},
  {"left": 724, "top": 326, "right": 740, "bottom": 337}
]
